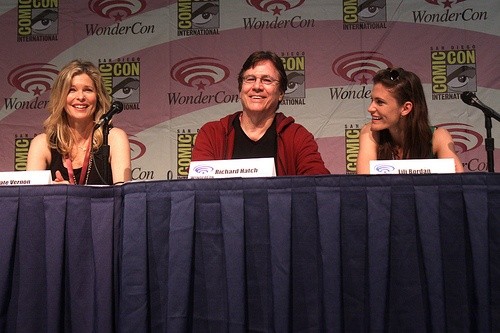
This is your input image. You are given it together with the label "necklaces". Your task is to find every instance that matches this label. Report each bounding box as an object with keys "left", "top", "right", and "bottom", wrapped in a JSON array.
[{"left": 75, "top": 145, "right": 88, "bottom": 151}]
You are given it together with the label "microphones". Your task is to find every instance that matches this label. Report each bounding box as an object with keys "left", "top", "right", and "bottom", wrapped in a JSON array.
[
  {"left": 94, "top": 101, "right": 123, "bottom": 129},
  {"left": 461, "top": 91, "right": 500, "bottom": 122}
]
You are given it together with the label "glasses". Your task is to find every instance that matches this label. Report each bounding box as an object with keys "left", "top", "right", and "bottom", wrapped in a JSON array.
[
  {"left": 242, "top": 77, "right": 280, "bottom": 85},
  {"left": 386, "top": 67, "right": 400, "bottom": 81}
]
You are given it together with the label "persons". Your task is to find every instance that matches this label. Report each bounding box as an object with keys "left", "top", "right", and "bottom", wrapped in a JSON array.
[
  {"left": 355, "top": 67, "right": 465, "bottom": 174},
  {"left": 187, "top": 51, "right": 331, "bottom": 176},
  {"left": 26, "top": 60, "right": 132, "bottom": 186}
]
